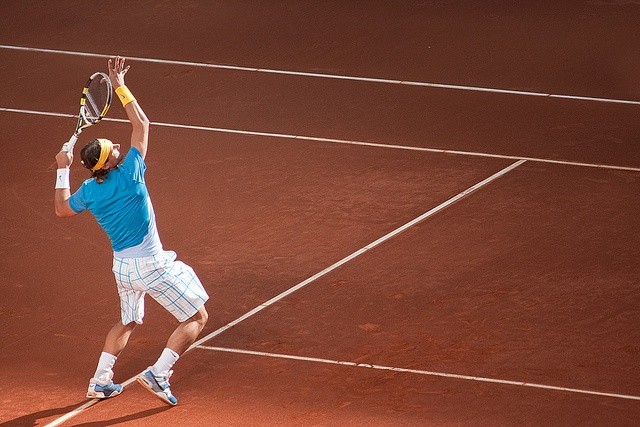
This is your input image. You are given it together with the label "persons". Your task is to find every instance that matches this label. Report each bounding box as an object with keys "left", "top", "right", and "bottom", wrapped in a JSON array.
[{"left": 55, "top": 55, "right": 209, "bottom": 405}]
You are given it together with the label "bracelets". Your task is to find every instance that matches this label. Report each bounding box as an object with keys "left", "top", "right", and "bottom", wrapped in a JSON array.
[
  {"left": 114, "top": 84, "right": 136, "bottom": 107},
  {"left": 55, "top": 168, "right": 70, "bottom": 189}
]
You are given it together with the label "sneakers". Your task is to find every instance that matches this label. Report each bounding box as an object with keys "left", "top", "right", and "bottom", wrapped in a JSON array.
[
  {"left": 86, "top": 377, "right": 123, "bottom": 399},
  {"left": 136, "top": 367, "right": 177, "bottom": 406}
]
